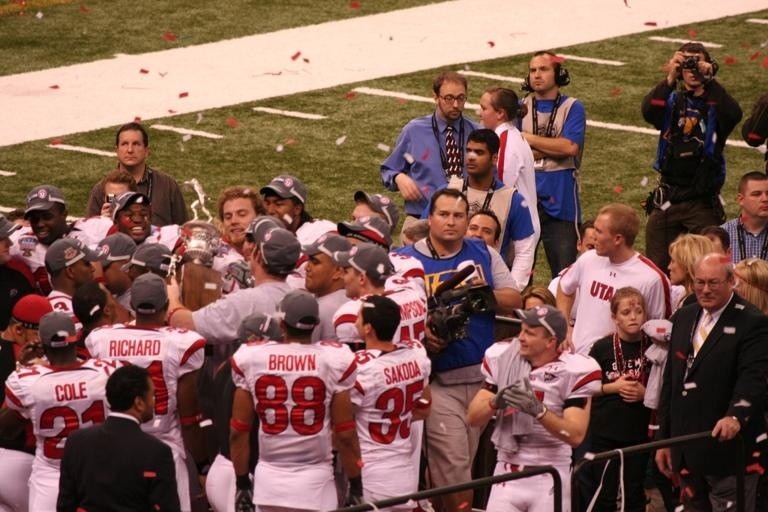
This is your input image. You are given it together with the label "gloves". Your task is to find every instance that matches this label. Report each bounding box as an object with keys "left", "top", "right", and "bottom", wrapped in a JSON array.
[
  {"left": 234, "top": 472, "right": 255, "bottom": 511},
  {"left": 489, "top": 377, "right": 547, "bottom": 421},
  {"left": 343, "top": 475, "right": 364, "bottom": 508}
]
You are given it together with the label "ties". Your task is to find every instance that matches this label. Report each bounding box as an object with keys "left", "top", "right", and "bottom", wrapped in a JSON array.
[
  {"left": 445, "top": 126, "right": 463, "bottom": 175},
  {"left": 692, "top": 312, "right": 714, "bottom": 360}
]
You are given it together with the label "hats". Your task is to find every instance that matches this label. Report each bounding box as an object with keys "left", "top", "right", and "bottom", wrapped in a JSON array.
[
  {"left": 241, "top": 174, "right": 399, "bottom": 340},
  {"left": 0, "top": 185, "right": 172, "bottom": 349},
  {"left": 513, "top": 304, "right": 568, "bottom": 343}
]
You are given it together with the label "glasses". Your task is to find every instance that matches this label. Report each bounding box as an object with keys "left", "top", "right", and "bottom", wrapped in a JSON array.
[
  {"left": 691, "top": 277, "right": 729, "bottom": 291},
  {"left": 439, "top": 94, "right": 468, "bottom": 104}
]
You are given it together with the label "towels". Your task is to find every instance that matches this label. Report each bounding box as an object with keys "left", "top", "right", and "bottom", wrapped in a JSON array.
[{"left": 491, "top": 336, "right": 537, "bottom": 457}]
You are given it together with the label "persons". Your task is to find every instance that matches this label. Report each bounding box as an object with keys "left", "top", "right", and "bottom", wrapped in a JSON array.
[{"left": 1, "top": 43, "right": 768, "bottom": 511}]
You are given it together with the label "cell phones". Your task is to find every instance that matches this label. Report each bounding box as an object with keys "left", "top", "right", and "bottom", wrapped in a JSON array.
[{"left": 105, "top": 194, "right": 116, "bottom": 202}]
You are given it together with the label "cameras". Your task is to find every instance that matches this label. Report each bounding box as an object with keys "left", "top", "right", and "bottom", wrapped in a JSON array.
[{"left": 680, "top": 55, "right": 697, "bottom": 70}]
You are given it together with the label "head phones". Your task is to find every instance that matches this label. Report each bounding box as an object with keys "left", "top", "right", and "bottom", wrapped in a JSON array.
[{"left": 521, "top": 50, "right": 569, "bottom": 89}]
